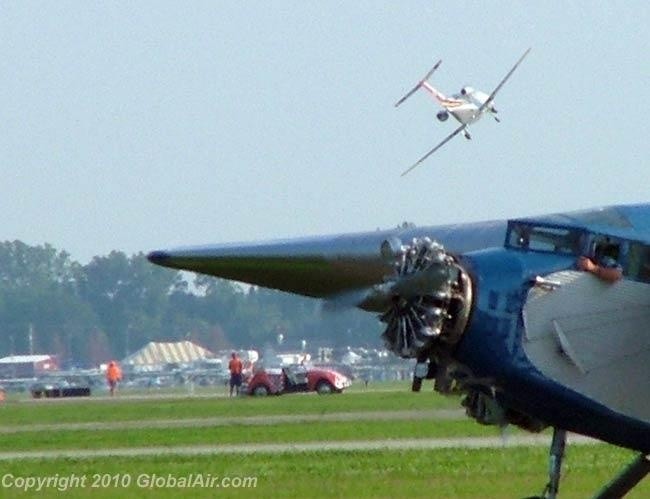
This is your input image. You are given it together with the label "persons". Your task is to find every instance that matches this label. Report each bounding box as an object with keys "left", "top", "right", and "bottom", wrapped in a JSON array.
[
  {"left": 228, "top": 352, "right": 243, "bottom": 398},
  {"left": 107, "top": 361, "right": 121, "bottom": 396},
  {"left": 577, "top": 234, "right": 623, "bottom": 280}
]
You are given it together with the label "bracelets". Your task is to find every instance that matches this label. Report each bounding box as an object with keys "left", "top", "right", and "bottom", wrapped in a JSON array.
[{"left": 591, "top": 264, "right": 598, "bottom": 273}]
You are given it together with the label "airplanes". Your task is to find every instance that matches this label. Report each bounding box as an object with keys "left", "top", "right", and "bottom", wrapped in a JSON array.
[
  {"left": 394, "top": 46, "right": 533, "bottom": 177},
  {"left": 147, "top": 218, "right": 650, "bottom": 499}
]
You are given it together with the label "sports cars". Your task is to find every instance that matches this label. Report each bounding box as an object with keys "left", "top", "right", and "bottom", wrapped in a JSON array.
[{"left": 247, "top": 363, "right": 352, "bottom": 397}]
